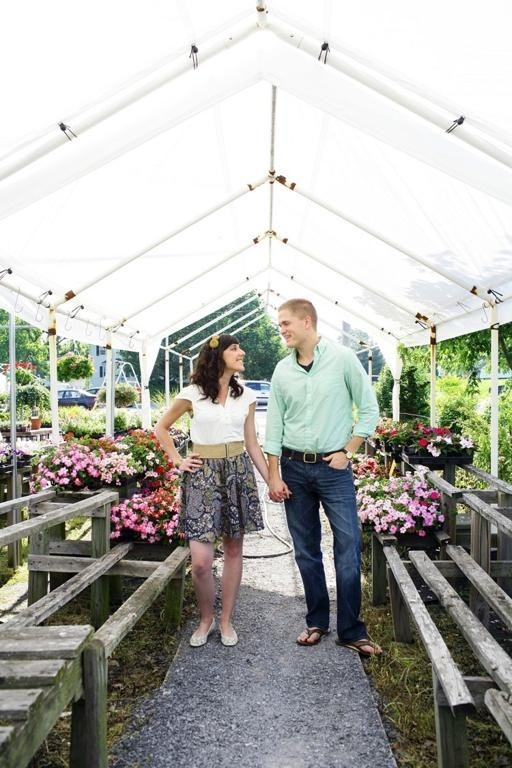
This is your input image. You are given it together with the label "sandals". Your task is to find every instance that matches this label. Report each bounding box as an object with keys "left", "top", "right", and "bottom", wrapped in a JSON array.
[
  {"left": 296, "top": 628, "right": 330, "bottom": 646},
  {"left": 334, "top": 638, "right": 379, "bottom": 655}
]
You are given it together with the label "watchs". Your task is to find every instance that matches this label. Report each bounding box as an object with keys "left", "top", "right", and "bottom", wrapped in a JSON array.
[{"left": 341, "top": 449, "right": 354, "bottom": 460}]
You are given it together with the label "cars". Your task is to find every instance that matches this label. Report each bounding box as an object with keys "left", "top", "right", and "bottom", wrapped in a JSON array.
[
  {"left": 56, "top": 389, "right": 97, "bottom": 409},
  {"left": 239, "top": 380, "right": 272, "bottom": 407}
]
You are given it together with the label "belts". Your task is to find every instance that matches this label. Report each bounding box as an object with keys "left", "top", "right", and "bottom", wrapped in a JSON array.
[{"left": 282, "top": 446, "right": 341, "bottom": 464}]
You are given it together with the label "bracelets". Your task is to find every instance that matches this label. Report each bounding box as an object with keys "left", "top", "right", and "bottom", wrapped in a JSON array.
[{"left": 175, "top": 459, "right": 184, "bottom": 469}]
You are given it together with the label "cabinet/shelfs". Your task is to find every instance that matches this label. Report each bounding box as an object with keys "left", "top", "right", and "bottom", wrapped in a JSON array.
[
  {"left": 370, "top": 464, "right": 511, "bottom": 768},
  {"left": 0, "top": 483, "right": 190, "bottom": 767}
]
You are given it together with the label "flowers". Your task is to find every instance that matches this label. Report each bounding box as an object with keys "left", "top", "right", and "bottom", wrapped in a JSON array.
[
  {"left": 0, "top": 416, "right": 193, "bottom": 547},
  {"left": 347, "top": 417, "right": 480, "bottom": 534}
]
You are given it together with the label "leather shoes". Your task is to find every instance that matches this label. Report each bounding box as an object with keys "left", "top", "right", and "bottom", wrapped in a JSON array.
[
  {"left": 219, "top": 624, "right": 238, "bottom": 646},
  {"left": 190, "top": 617, "right": 216, "bottom": 647}
]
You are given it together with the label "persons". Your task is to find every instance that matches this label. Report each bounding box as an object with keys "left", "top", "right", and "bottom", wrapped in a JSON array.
[
  {"left": 153, "top": 334, "right": 293, "bottom": 647},
  {"left": 263, "top": 298, "right": 385, "bottom": 657}
]
